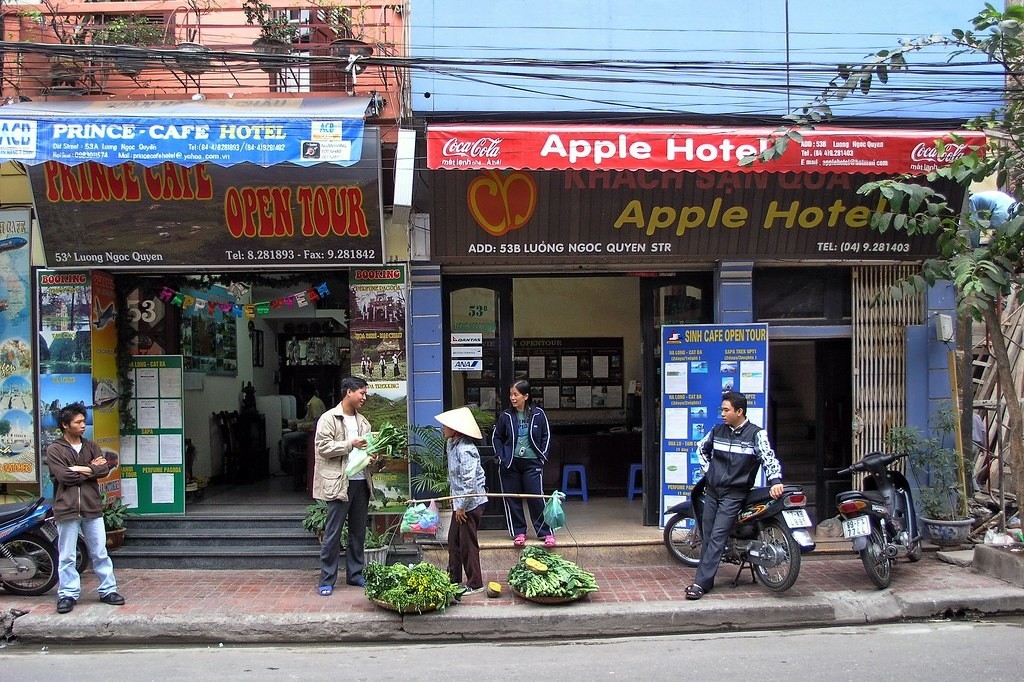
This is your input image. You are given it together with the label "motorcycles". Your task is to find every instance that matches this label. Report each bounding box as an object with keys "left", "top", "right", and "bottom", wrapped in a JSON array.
[
  {"left": 836, "top": 451, "right": 923, "bottom": 589},
  {"left": 0, "top": 496, "right": 90, "bottom": 597},
  {"left": 662, "top": 465, "right": 816, "bottom": 592}
]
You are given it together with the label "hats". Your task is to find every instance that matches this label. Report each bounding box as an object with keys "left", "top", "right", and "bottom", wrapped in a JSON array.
[
  {"left": 434, "top": 407, "right": 483, "bottom": 439},
  {"left": 183, "top": 316, "right": 189, "bottom": 322},
  {"left": 367, "top": 356, "right": 370, "bottom": 360},
  {"left": 392, "top": 356, "right": 395, "bottom": 359},
  {"left": 206, "top": 320, "right": 215, "bottom": 329},
  {"left": 362, "top": 357, "right": 364, "bottom": 360},
  {"left": 394, "top": 354, "right": 397, "bottom": 356},
  {"left": 380, "top": 356, "right": 383, "bottom": 359}
]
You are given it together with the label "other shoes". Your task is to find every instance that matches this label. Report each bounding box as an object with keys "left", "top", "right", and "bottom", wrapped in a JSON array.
[
  {"left": 275, "top": 471, "right": 291, "bottom": 476},
  {"left": 462, "top": 584, "right": 484, "bottom": 595}
]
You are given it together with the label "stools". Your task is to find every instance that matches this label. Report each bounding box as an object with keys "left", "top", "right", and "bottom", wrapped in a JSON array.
[
  {"left": 628, "top": 464, "right": 646, "bottom": 501},
  {"left": 561, "top": 464, "right": 588, "bottom": 503}
]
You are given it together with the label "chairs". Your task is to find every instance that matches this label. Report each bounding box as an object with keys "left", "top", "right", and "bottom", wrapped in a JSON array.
[{"left": 212, "top": 410, "right": 271, "bottom": 485}]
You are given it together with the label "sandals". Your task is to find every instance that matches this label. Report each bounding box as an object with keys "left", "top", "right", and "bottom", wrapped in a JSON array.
[
  {"left": 356, "top": 577, "right": 366, "bottom": 587},
  {"left": 684, "top": 584, "right": 704, "bottom": 599},
  {"left": 545, "top": 534, "right": 556, "bottom": 546},
  {"left": 318, "top": 585, "right": 332, "bottom": 596},
  {"left": 514, "top": 534, "right": 526, "bottom": 547}
]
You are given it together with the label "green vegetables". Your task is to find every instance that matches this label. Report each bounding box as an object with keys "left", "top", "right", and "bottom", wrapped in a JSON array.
[
  {"left": 363, "top": 561, "right": 468, "bottom": 613},
  {"left": 508, "top": 546, "right": 599, "bottom": 598}
]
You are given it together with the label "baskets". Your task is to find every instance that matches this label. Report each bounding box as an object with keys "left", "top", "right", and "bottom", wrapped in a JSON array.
[
  {"left": 508, "top": 583, "right": 588, "bottom": 604},
  {"left": 371, "top": 597, "right": 437, "bottom": 612}
]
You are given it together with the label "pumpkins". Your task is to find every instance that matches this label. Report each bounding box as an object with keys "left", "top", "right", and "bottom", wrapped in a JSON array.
[
  {"left": 487, "top": 581, "right": 502, "bottom": 597},
  {"left": 525, "top": 558, "right": 548, "bottom": 575}
]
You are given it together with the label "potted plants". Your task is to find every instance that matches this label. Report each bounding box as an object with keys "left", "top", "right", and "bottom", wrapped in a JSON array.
[
  {"left": 242, "top": 0, "right": 305, "bottom": 73},
  {"left": 91, "top": 12, "right": 170, "bottom": 77},
  {"left": 41, "top": 0, "right": 90, "bottom": 82},
  {"left": 307, "top": 0, "right": 397, "bottom": 75},
  {"left": 881, "top": 400, "right": 975, "bottom": 546},
  {"left": 364, "top": 523, "right": 399, "bottom": 566},
  {"left": 102, "top": 493, "right": 131, "bottom": 550},
  {"left": 302, "top": 499, "right": 349, "bottom": 552},
  {"left": 174, "top": 0, "right": 222, "bottom": 74}
]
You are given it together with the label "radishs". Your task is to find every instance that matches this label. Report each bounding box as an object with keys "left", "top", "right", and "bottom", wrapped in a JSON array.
[{"left": 345, "top": 451, "right": 372, "bottom": 476}]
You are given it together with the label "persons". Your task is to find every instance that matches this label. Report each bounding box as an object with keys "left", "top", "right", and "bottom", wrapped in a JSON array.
[
  {"left": 433, "top": 407, "right": 483, "bottom": 596},
  {"left": 46, "top": 401, "right": 125, "bottom": 614},
  {"left": 313, "top": 377, "right": 378, "bottom": 596},
  {"left": 288, "top": 336, "right": 300, "bottom": 366},
  {"left": 275, "top": 380, "right": 327, "bottom": 477},
  {"left": 241, "top": 380, "right": 256, "bottom": 408},
  {"left": 379, "top": 356, "right": 385, "bottom": 378},
  {"left": 392, "top": 354, "right": 400, "bottom": 376},
  {"left": 360, "top": 356, "right": 374, "bottom": 376},
  {"left": 491, "top": 380, "right": 556, "bottom": 547},
  {"left": 685, "top": 392, "right": 785, "bottom": 600},
  {"left": 972, "top": 406, "right": 987, "bottom": 492}
]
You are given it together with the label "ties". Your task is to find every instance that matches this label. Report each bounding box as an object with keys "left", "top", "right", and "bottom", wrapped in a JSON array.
[{"left": 186, "top": 366, "right": 188, "bottom": 369}]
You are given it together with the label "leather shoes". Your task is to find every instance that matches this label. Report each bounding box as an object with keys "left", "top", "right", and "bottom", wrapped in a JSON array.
[
  {"left": 100, "top": 592, "right": 125, "bottom": 604},
  {"left": 57, "top": 596, "right": 76, "bottom": 613}
]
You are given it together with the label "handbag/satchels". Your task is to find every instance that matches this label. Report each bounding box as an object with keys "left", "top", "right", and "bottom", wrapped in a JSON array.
[
  {"left": 399, "top": 499, "right": 437, "bottom": 534},
  {"left": 543, "top": 490, "right": 566, "bottom": 529},
  {"left": 346, "top": 434, "right": 374, "bottom": 477}
]
